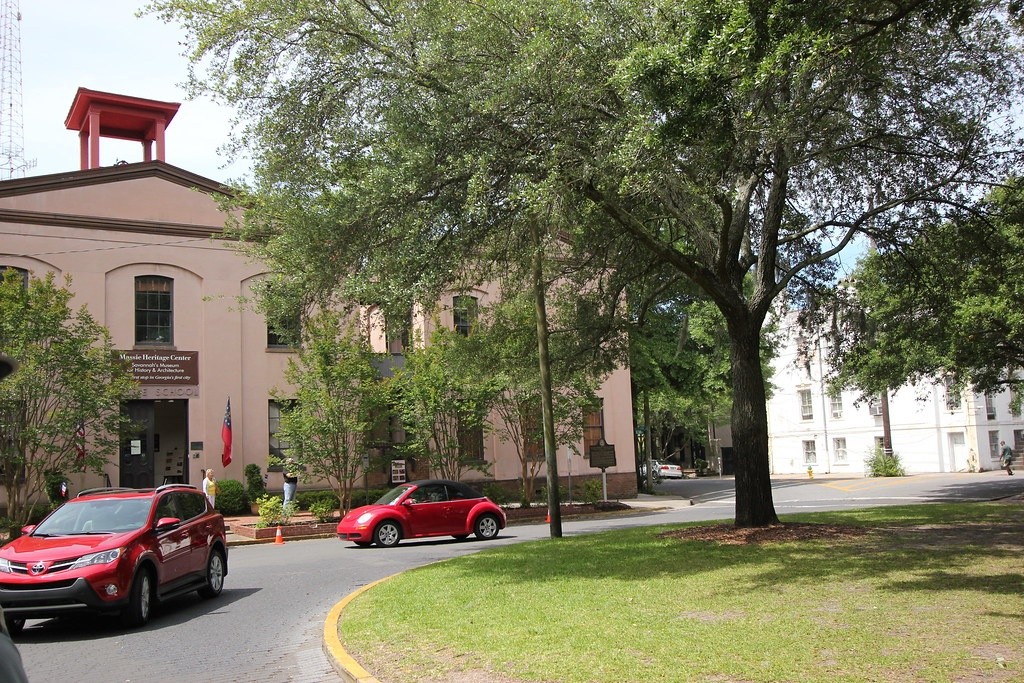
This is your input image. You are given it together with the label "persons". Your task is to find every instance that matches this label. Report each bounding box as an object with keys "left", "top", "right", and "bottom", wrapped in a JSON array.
[
  {"left": 203, "top": 468, "right": 219, "bottom": 509},
  {"left": 280, "top": 458, "right": 298, "bottom": 518},
  {"left": 998, "top": 440, "right": 1015, "bottom": 476}
]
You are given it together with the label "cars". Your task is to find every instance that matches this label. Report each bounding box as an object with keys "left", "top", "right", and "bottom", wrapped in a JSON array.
[
  {"left": 639, "top": 458, "right": 683, "bottom": 479},
  {"left": 337, "top": 479, "right": 508, "bottom": 548}
]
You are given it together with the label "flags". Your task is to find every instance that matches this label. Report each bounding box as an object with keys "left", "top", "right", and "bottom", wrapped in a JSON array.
[{"left": 221, "top": 395, "right": 232, "bottom": 468}]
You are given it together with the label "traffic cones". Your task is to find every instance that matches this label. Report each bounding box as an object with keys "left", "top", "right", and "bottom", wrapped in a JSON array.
[
  {"left": 544, "top": 508, "right": 550, "bottom": 523},
  {"left": 272, "top": 525, "right": 285, "bottom": 545}
]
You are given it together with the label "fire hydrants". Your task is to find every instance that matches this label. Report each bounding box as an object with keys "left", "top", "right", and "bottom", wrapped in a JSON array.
[{"left": 806, "top": 465, "right": 814, "bottom": 480}]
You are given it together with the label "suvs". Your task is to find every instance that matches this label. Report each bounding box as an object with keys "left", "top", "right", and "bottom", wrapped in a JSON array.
[{"left": 0, "top": 486, "right": 230, "bottom": 627}]
